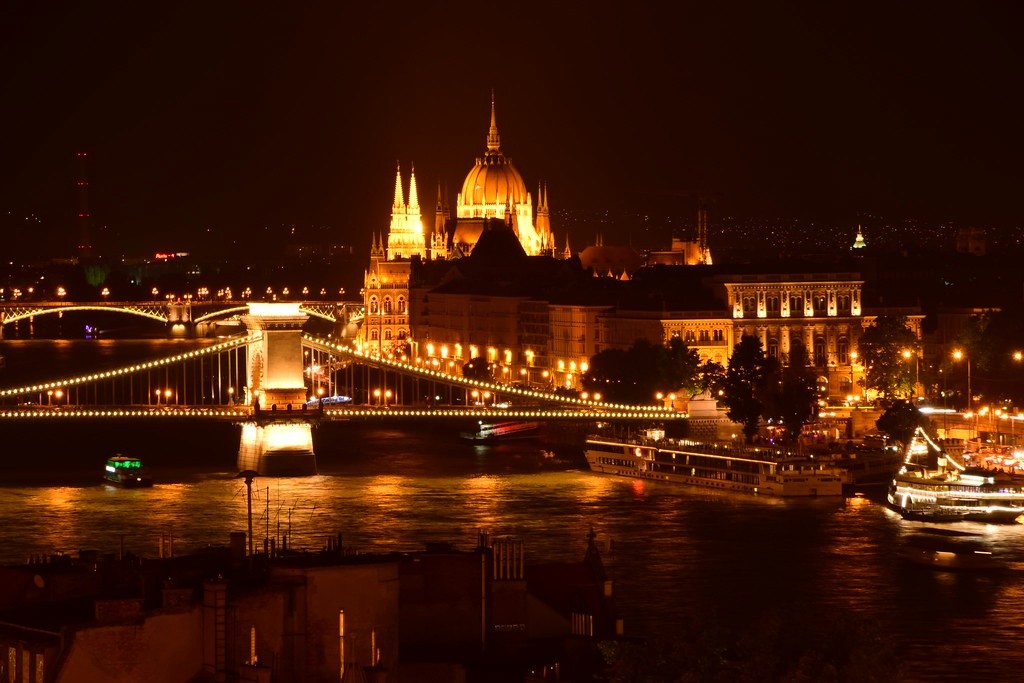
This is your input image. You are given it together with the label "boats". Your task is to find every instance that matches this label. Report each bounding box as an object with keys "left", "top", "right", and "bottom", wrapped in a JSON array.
[
  {"left": 582, "top": 427, "right": 843, "bottom": 497},
  {"left": 961, "top": 445, "right": 1024, "bottom": 467},
  {"left": 888, "top": 467, "right": 1024, "bottom": 525},
  {"left": 101, "top": 451, "right": 156, "bottom": 485},
  {"left": 458, "top": 419, "right": 544, "bottom": 442}
]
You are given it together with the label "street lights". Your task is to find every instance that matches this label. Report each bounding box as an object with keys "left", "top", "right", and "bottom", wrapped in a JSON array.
[
  {"left": 503, "top": 349, "right": 513, "bottom": 384},
  {"left": 470, "top": 345, "right": 479, "bottom": 379},
  {"left": 454, "top": 343, "right": 463, "bottom": 376},
  {"left": 524, "top": 349, "right": 534, "bottom": 389},
  {"left": 426, "top": 342, "right": 434, "bottom": 371},
  {"left": 489, "top": 348, "right": 497, "bottom": 383},
  {"left": 440, "top": 345, "right": 449, "bottom": 374}
]
